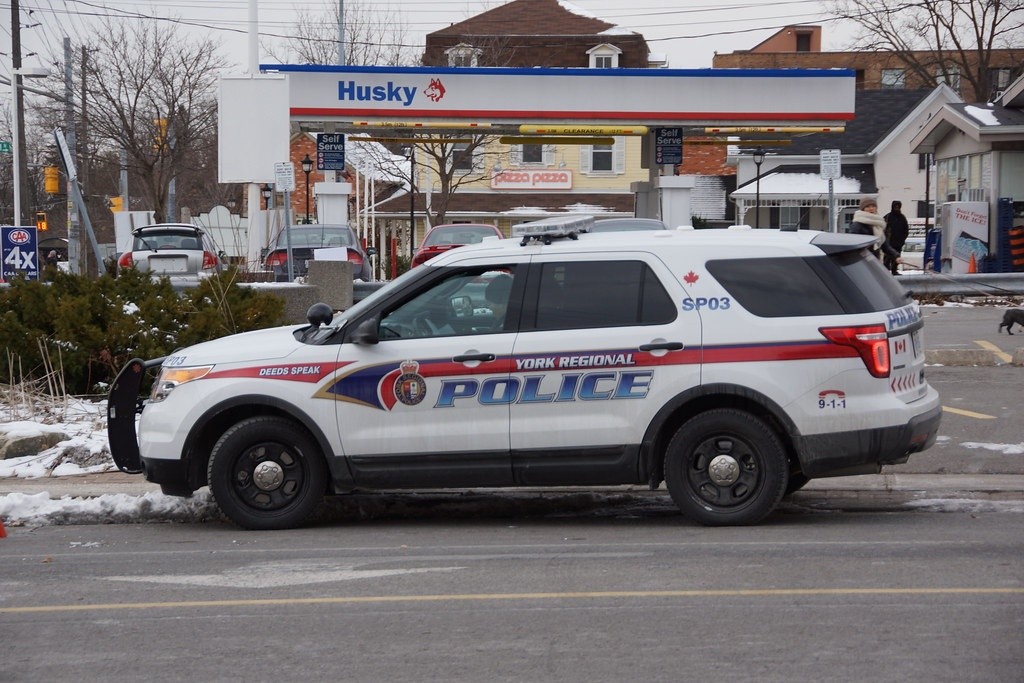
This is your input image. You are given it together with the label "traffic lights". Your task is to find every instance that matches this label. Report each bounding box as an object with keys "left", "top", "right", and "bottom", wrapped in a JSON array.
[
  {"left": 37, "top": 213, "right": 52, "bottom": 233},
  {"left": 108, "top": 198, "right": 124, "bottom": 215}
]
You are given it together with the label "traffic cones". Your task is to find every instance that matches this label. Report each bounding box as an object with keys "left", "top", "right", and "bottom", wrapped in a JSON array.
[{"left": 966, "top": 254, "right": 977, "bottom": 275}]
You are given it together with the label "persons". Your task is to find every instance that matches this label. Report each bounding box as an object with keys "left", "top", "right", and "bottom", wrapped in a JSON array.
[
  {"left": 881, "top": 199, "right": 911, "bottom": 273},
  {"left": 482, "top": 274, "right": 513, "bottom": 328},
  {"left": 45, "top": 250, "right": 57, "bottom": 281},
  {"left": 850, "top": 193, "right": 904, "bottom": 266}
]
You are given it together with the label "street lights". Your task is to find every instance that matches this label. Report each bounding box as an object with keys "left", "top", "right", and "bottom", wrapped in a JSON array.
[
  {"left": 403, "top": 143, "right": 419, "bottom": 262},
  {"left": 301, "top": 153, "right": 316, "bottom": 225},
  {"left": 752, "top": 145, "right": 768, "bottom": 230}
]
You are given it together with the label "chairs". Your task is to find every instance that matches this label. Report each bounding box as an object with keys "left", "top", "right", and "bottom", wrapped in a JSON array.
[
  {"left": 328, "top": 237, "right": 346, "bottom": 245},
  {"left": 549, "top": 272, "right": 592, "bottom": 326},
  {"left": 436, "top": 240, "right": 453, "bottom": 246},
  {"left": 140, "top": 240, "right": 158, "bottom": 249},
  {"left": 181, "top": 239, "right": 196, "bottom": 249}
]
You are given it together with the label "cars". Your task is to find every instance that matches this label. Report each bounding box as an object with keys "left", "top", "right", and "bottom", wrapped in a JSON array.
[
  {"left": 102, "top": 252, "right": 124, "bottom": 278},
  {"left": 262, "top": 223, "right": 378, "bottom": 285},
  {"left": 39, "top": 237, "right": 69, "bottom": 278},
  {"left": 411, "top": 222, "right": 513, "bottom": 275}
]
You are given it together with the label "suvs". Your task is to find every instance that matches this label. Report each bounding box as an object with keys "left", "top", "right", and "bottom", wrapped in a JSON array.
[
  {"left": 104, "top": 206, "right": 943, "bottom": 531},
  {"left": 116, "top": 221, "right": 226, "bottom": 290}
]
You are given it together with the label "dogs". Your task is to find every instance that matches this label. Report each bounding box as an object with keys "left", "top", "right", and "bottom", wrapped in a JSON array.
[{"left": 997, "top": 302, "right": 1024, "bottom": 336}]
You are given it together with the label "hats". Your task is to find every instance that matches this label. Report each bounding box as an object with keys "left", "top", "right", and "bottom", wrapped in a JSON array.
[
  {"left": 860, "top": 197, "right": 877, "bottom": 211},
  {"left": 485, "top": 274, "right": 513, "bottom": 305}
]
